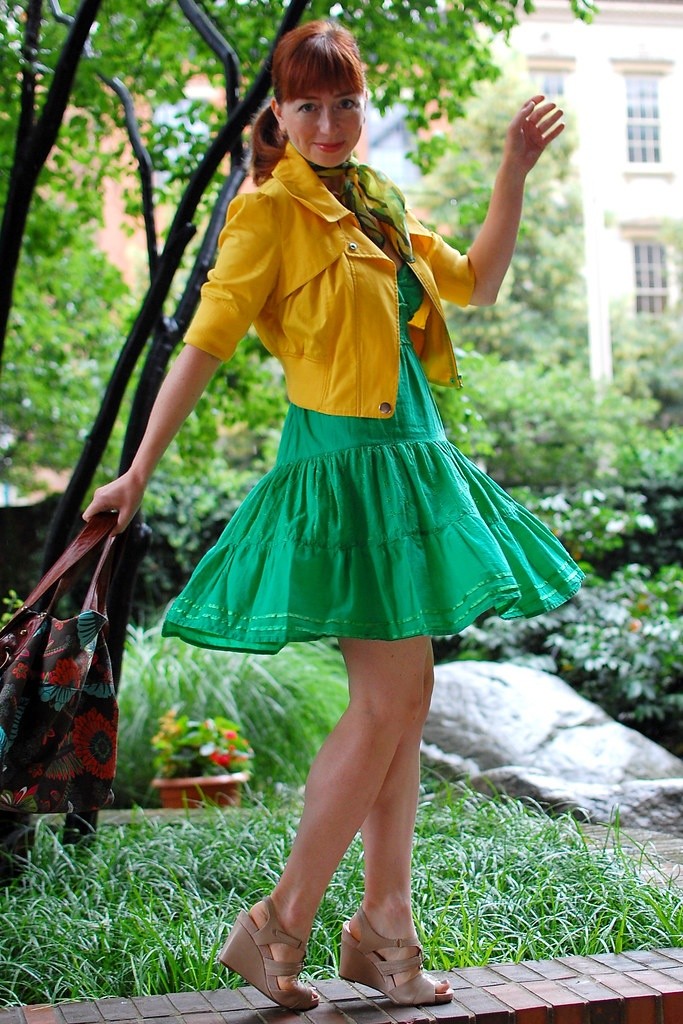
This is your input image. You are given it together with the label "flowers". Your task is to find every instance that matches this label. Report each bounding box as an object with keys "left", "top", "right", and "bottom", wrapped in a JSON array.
[{"left": 150, "top": 699, "right": 256, "bottom": 778}]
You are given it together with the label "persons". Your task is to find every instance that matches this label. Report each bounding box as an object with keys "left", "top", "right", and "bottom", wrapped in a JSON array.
[{"left": 81, "top": 19, "right": 566, "bottom": 1009}]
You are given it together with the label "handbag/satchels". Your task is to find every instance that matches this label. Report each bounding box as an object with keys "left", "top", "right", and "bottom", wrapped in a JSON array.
[{"left": 0, "top": 512, "right": 119, "bottom": 814}]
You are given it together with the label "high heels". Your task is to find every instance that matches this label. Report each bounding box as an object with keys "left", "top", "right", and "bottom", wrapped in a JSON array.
[
  {"left": 218, "top": 897, "right": 319, "bottom": 1010},
  {"left": 338, "top": 906, "right": 452, "bottom": 1006}
]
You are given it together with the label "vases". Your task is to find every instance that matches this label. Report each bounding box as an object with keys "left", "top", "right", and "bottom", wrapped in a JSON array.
[{"left": 151, "top": 771, "right": 251, "bottom": 807}]
